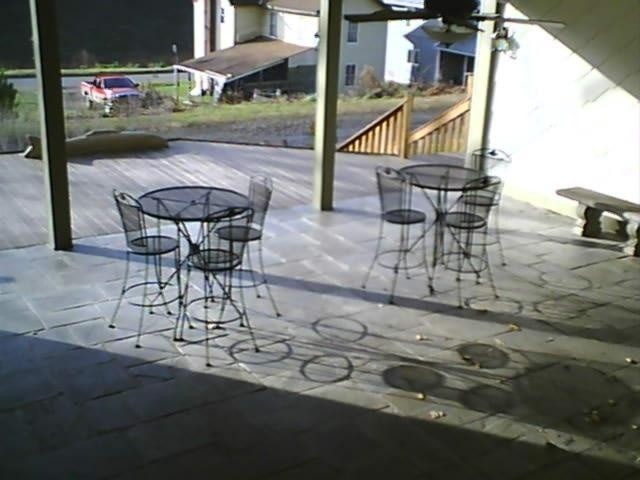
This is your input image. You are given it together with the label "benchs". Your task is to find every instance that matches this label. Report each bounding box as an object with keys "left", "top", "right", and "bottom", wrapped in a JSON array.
[{"left": 556, "top": 186, "right": 639, "bottom": 257}]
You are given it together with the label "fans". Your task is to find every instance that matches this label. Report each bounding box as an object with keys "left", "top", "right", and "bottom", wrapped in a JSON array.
[{"left": 342, "top": 0, "right": 564, "bottom": 27}]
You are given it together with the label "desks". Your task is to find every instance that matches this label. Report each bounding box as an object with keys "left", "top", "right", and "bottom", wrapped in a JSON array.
[
  {"left": 398, "top": 161, "right": 491, "bottom": 292},
  {"left": 135, "top": 184, "right": 253, "bottom": 329}
]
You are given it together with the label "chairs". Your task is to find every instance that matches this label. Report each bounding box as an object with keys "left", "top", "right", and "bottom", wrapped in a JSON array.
[
  {"left": 452, "top": 147, "right": 509, "bottom": 265},
  {"left": 438, "top": 171, "right": 501, "bottom": 311},
  {"left": 369, "top": 164, "right": 435, "bottom": 304},
  {"left": 107, "top": 190, "right": 195, "bottom": 349},
  {"left": 204, "top": 171, "right": 289, "bottom": 318},
  {"left": 174, "top": 202, "right": 260, "bottom": 367}
]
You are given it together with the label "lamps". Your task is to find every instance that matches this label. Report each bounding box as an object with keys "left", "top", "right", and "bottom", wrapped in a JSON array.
[{"left": 420, "top": 20, "right": 478, "bottom": 49}]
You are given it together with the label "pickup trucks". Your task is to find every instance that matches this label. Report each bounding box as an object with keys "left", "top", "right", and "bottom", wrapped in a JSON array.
[{"left": 80, "top": 75, "right": 149, "bottom": 116}]
